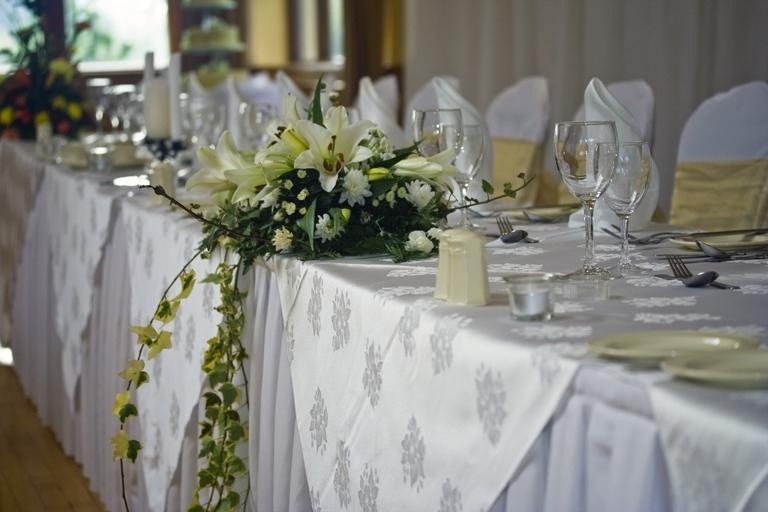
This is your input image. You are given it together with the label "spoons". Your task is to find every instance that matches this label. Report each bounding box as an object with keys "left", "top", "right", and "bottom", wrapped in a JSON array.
[
  {"left": 654, "top": 272, "right": 719, "bottom": 288},
  {"left": 695, "top": 240, "right": 765, "bottom": 257},
  {"left": 482, "top": 230, "right": 528, "bottom": 244}
]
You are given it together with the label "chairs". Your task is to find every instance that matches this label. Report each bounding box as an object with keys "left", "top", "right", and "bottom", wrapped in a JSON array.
[{"left": 476, "top": 74, "right": 768, "bottom": 247}]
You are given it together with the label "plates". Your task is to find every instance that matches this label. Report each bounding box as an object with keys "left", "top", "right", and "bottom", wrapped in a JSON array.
[
  {"left": 670, "top": 230, "right": 768, "bottom": 251},
  {"left": 590, "top": 331, "right": 760, "bottom": 362},
  {"left": 660, "top": 347, "right": 768, "bottom": 390}
]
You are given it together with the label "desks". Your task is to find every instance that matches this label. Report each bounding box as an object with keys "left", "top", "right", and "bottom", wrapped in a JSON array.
[{"left": 1, "top": 141, "right": 765, "bottom": 510}]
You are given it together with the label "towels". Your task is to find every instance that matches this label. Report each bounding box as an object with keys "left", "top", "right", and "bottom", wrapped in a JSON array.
[
  {"left": 432, "top": 75, "right": 493, "bottom": 184},
  {"left": 358, "top": 72, "right": 399, "bottom": 136}
]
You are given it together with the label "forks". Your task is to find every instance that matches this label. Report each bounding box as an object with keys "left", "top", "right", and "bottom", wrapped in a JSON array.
[
  {"left": 667, "top": 255, "right": 740, "bottom": 290},
  {"left": 495, "top": 216, "right": 539, "bottom": 244},
  {"left": 600, "top": 224, "right": 768, "bottom": 244}
]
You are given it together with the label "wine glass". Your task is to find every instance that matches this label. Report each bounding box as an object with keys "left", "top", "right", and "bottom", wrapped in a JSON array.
[
  {"left": 88, "top": 77, "right": 273, "bottom": 183},
  {"left": 595, "top": 142, "right": 656, "bottom": 281},
  {"left": 413, "top": 107, "right": 490, "bottom": 231},
  {"left": 554, "top": 121, "right": 623, "bottom": 283}
]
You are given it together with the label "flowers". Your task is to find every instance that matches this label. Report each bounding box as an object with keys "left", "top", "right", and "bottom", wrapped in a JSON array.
[
  {"left": 0, "top": 17, "right": 97, "bottom": 142},
  {"left": 105, "top": 95, "right": 458, "bottom": 510}
]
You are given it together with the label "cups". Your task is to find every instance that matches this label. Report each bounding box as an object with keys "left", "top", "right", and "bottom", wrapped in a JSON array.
[
  {"left": 503, "top": 272, "right": 555, "bottom": 321},
  {"left": 434, "top": 222, "right": 494, "bottom": 307},
  {"left": 148, "top": 156, "right": 176, "bottom": 205}
]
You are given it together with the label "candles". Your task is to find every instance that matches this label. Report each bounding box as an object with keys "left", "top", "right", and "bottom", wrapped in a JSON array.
[{"left": 144, "top": 76, "right": 170, "bottom": 140}]
